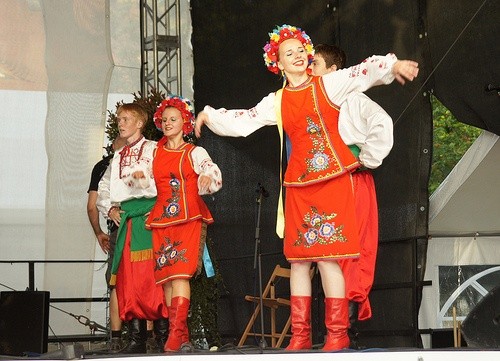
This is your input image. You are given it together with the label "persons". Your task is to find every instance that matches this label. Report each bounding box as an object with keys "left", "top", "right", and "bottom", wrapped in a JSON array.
[
  {"left": 195, "top": 24, "right": 419, "bottom": 351},
  {"left": 96, "top": 103, "right": 170, "bottom": 354},
  {"left": 86, "top": 135, "right": 161, "bottom": 354},
  {"left": 122, "top": 95, "right": 222, "bottom": 353},
  {"left": 310, "top": 43, "right": 394, "bottom": 350}
]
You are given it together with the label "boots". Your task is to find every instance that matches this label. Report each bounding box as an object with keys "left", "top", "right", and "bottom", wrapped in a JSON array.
[
  {"left": 321, "top": 296, "right": 350, "bottom": 351},
  {"left": 309, "top": 297, "right": 324, "bottom": 347},
  {"left": 283, "top": 296, "right": 312, "bottom": 350},
  {"left": 348, "top": 300, "right": 366, "bottom": 351},
  {"left": 162, "top": 297, "right": 194, "bottom": 353},
  {"left": 151, "top": 317, "right": 169, "bottom": 354},
  {"left": 119, "top": 316, "right": 148, "bottom": 354}
]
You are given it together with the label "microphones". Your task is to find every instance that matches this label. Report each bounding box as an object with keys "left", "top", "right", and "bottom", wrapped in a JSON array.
[
  {"left": 258, "top": 182, "right": 269, "bottom": 198},
  {"left": 485, "top": 83, "right": 500, "bottom": 93}
]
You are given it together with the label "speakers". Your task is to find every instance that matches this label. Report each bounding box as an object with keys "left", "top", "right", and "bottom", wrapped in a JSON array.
[
  {"left": 460, "top": 284, "right": 500, "bottom": 348},
  {"left": 0, "top": 290, "right": 50, "bottom": 357}
]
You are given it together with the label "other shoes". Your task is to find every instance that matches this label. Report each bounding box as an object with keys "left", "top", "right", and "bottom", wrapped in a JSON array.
[{"left": 108, "top": 337, "right": 128, "bottom": 353}]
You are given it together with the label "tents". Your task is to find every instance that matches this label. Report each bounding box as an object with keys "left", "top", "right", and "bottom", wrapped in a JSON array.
[{"left": 419, "top": 128, "right": 500, "bottom": 349}]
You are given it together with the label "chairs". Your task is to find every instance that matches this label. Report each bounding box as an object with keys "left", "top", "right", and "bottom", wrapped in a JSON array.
[{"left": 238, "top": 264, "right": 318, "bottom": 348}]
